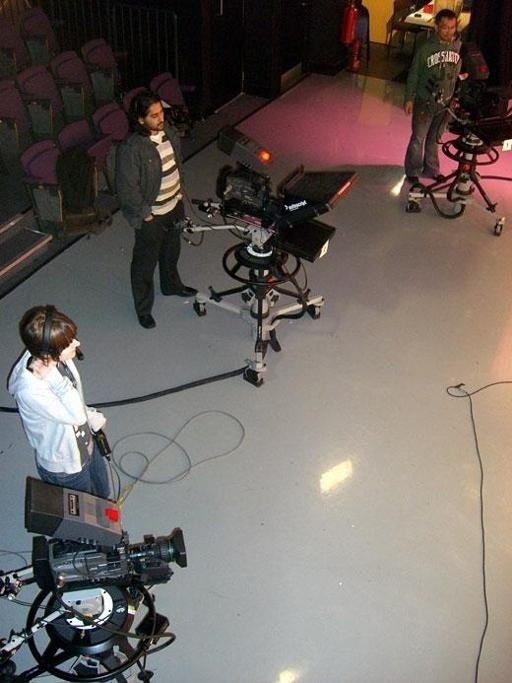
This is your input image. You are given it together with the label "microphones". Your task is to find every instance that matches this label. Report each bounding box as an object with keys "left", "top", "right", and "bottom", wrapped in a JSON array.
[{"left": 76, "top": 346, "right": 85, "bottom": 360}]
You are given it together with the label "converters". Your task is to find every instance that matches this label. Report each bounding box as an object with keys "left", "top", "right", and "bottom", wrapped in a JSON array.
[{"left": 95, "top": 429, "right": 111, "bottom": 456}]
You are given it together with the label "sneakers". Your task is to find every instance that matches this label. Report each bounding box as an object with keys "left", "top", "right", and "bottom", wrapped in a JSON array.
[
  {"left": 435, "top": 175, "right": 444, "bottom": 179},
  {"left": 178, "top": 285, "right": 197, "bottom": 296},
  {"left": 410, "top": 177, "right": 419, "bottom": 184},
  {"left": 138, "top": 314, "right": 156, "bottom": 328}
]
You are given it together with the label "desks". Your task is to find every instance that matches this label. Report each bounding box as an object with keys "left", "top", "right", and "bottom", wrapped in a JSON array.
[{"left": 392, "top": 0, "right": 471, "bottom": 85}]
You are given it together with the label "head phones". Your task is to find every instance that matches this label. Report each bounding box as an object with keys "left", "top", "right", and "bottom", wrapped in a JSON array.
[{"left": 32, "top": 304, "right": 58, "bottom": 363}]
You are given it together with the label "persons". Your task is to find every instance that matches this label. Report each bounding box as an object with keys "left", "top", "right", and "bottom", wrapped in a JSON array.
[
  {"left": 4, "top": 301, "right": 111, "bottom": 505},
  {"left": 402, "top": 8, "right": 469, "bottom": 187},
  {"left": 114, "top": 90, "right": 198, "bottom": 330}
]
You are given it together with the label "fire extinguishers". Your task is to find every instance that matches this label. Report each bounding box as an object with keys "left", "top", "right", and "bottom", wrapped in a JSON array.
[{"left": 339, "top": 0, "right": 361, "bottom": 73}]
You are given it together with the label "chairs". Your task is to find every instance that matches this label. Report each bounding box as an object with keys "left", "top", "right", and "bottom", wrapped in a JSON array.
[
  {"left": 388, "top": 0, "right": 432, "bottom": 61},
  {"left": 0, "top": 0, "right": 185, "bottom": 186}
]
